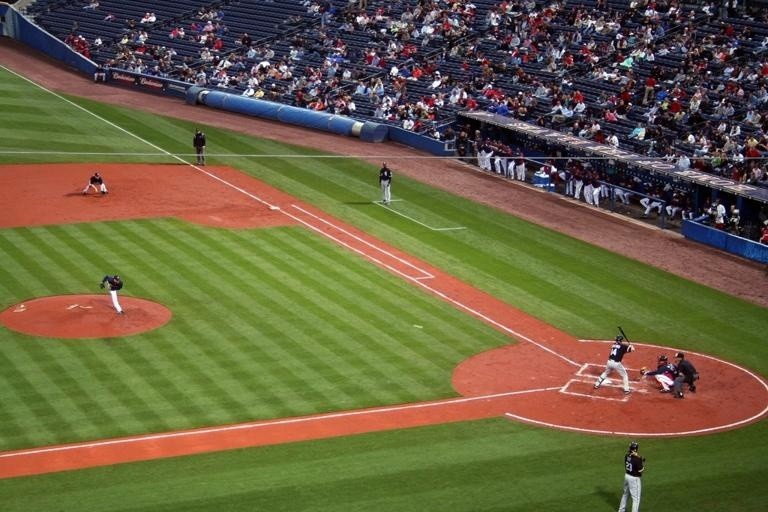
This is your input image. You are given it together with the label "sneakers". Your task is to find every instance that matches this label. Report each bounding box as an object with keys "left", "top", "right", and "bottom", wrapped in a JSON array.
[{"left": 659, "top": 384, "right": 696, "bottom": 398}]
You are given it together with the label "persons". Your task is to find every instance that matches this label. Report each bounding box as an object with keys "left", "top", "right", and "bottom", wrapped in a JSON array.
[
  {"left": 83, "top": 173, "right": 108, "bottom": 194},
  {"left": 639, "top": 355, "right": 678, "bottom": 393},
  {"left": 168, "top": 7, "right": 299, "bottom": 101},
  {"left": 591, "top": 335, "right": 635, "bottom": 394},
  {"left": 563, "top": 156, "right": 767, "bottom": 244},
  {"left": 193, "top": 128, "right": 206, "bottom": 165},
  {"left": 68, "top": 2, "right": 177, "bottom": 77},
  {"left": 379, "top": 161, "right": 393, "bottom": 204},
  {"left": 672, "top": 352, "right": 700, "bottom": 398},
  {"left": 617, "top": 443, "right": 645, "bottom": 512},
  {"left": 98, "top": 274, "right": 125, "bottom": 314},
  {"left": 294, "top": 1, "right": 767, "bottom": 187}
]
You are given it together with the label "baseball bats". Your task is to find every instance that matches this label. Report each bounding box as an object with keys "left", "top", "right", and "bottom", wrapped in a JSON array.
[{"left": 618, "top": 326, "right": 634, "bottom": 352}]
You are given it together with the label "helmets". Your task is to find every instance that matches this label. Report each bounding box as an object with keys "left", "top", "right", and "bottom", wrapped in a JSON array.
[
  {"left": 673, "top": 352, "right": 684, "bottom": 358},
  {"left": 630, "top": 442, "right": 638, "bottom": 451},
  {"left": 616, "top": 335, "right": 623, "bottom": 342},
  {"left": 656, "top": 354, "right": 668, "bottom": 364}
]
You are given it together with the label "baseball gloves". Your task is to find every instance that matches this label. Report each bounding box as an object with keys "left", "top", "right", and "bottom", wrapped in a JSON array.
[{"left": 639, "top": 366, "right": 648, "bottom": 375}]
